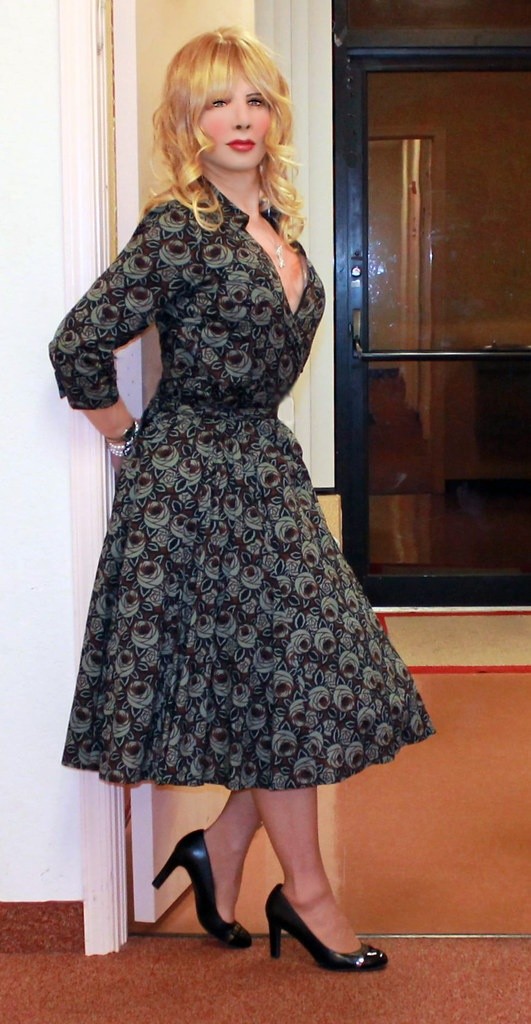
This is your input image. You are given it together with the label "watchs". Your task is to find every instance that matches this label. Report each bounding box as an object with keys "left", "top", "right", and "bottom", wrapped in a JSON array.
[{"left": 105, "top": 420, "right": 136, "bottom": 442}]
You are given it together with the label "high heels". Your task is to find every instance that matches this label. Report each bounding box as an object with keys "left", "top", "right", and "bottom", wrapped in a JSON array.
[
  {"left": 152, "top": 829, "right": 251, "bottom": 948},
  {"left": 265, "top": 883, "right": 388, "bottom": 971}
]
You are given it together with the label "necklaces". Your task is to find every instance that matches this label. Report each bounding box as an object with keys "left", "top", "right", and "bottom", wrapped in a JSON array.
[{"left": 268, "top": 239, "right": 286, "bottom": 269}]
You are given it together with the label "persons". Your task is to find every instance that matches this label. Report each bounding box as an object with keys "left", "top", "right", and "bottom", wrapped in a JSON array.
[{"left": 47, "top": 25, "right": 437, "bottom": 974}]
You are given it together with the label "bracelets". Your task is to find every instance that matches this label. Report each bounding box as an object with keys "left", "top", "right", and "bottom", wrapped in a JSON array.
[{"left": 109, "top": 421, "right": 139, "bottom": 457}]
